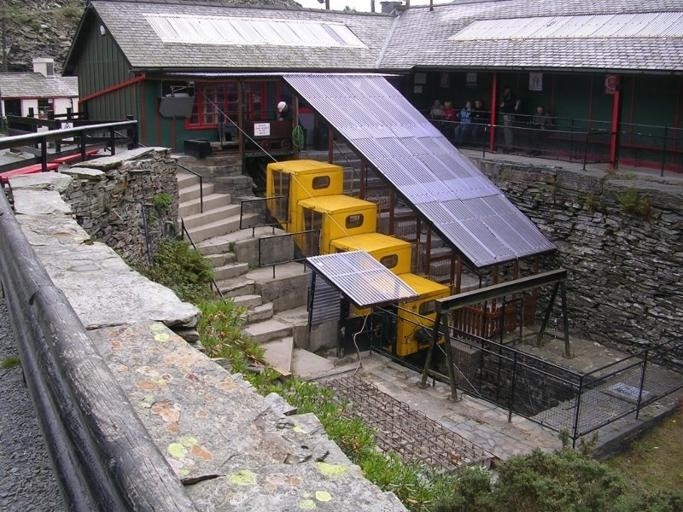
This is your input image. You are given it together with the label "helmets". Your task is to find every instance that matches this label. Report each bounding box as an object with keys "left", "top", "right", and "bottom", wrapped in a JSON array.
[{"left": 277, "top": 101, "right": 286, "bottom": 112}]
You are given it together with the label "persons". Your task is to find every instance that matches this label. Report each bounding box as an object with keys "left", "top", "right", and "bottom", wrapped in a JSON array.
[
  {"left": 429, "top": 87, "right": 549, "bottom": 156},
  {"left": 275, "top": 102, "right": 304, "bottom": 132}
]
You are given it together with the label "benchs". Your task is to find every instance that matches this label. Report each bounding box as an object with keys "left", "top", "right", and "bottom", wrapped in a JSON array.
[{"left": 0, "top": 149, "right": 100, "bottom": 188}]
